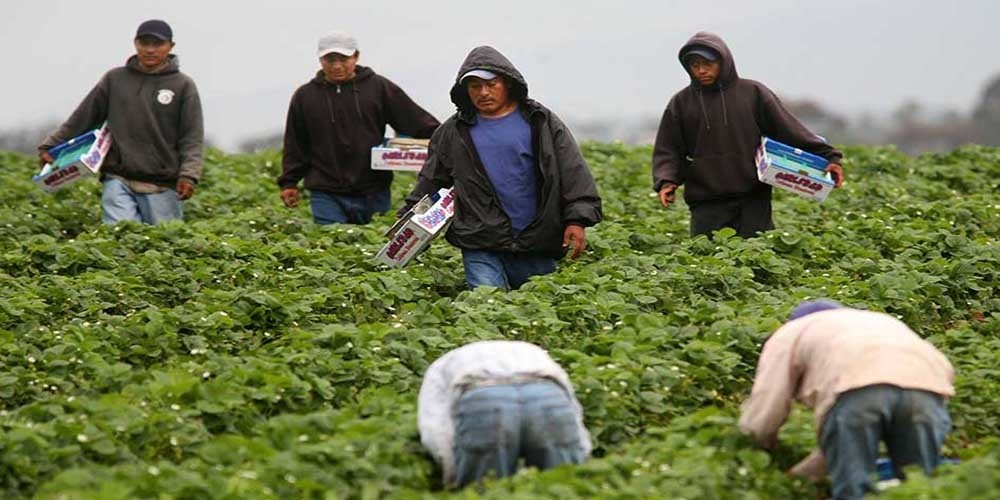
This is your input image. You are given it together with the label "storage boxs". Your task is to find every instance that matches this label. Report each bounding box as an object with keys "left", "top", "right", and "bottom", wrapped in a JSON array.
[
  {"left": 33, "top": 119, "right": 115, "bottom": 192},
  {"left": 371, "top": 136, "right": 434, "bottom": 171},
  {"left": 377, "top": 186, "right": 460, "bottom": 274},
  {"left": 756, "top": 137, "right": 834, "bottom": 200}
]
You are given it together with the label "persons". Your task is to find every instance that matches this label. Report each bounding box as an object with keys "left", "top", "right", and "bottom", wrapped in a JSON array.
[
  {"left": 737, "top": 298, "right": 955, "bottom": 500},
  {"left": 278, "top": 31, "right": 442, "bottom": 229},
  {"left": 38, "top": 22, "right": 203, "bottom": 227},
  {"left": 397, "top": 46, "right": 603, "bottom": 295},
  {"left": 651, "top": 31, "right": 844, "bottom": 237},
  {"left": 416, "top": 339, "right": 598, "bottom": 489}
]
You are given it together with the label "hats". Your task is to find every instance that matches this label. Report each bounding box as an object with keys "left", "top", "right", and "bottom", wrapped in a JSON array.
[
  {"left": 682, "top": 49, "right": 719, "bottom": 64},
  {"left": 316, "top": 33, "right": 360, "bottom": 57},
  {"left": 135, "top": 20, "right": 172, "bottom": 43},
  {"left": 459, "top": 70, "right": 498, "bottom": 85}
]
